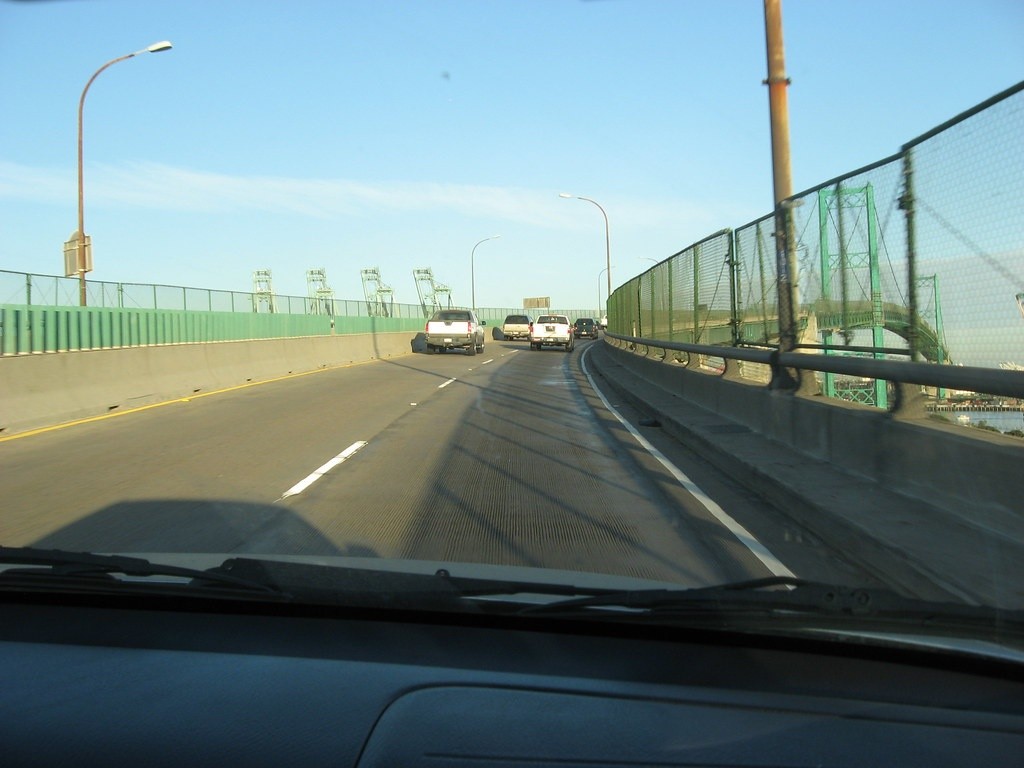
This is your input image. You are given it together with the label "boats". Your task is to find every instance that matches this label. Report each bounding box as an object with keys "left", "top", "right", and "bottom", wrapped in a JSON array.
[{"left": 956, "top": 415, "right": 969, "bottom": 420}]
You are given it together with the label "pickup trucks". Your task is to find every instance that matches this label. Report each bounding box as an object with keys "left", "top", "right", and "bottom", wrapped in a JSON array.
[
  {"left": 426, "top": 310, "right": 487, "bottom": 356},
  {"left": 528, "top": 315, "right": 578, "bottom": 352}
]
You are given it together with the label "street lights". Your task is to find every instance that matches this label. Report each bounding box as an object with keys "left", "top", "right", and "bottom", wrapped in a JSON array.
[
  {"left": 640, "top": 257, "right": 665, "bottom": 330},
  {"left": 598, "top": 266, "right": 616, "bottom": 331},
  {"left": 472, "top": 235, "right": 501, "bottom": 312},
  {"left": 80, "top": 40, "right": 173, "bottom": 306},
  {"left": 559, "top": 193, "right": 610, "bottom": 299}
]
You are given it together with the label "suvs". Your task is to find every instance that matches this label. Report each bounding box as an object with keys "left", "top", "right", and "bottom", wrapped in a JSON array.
[
  {"left": 573, "top": 315, "right": 608, "bottom": 339},
  {"left": 502, "top": 314, "right": 535, "bottom": 341}
]
